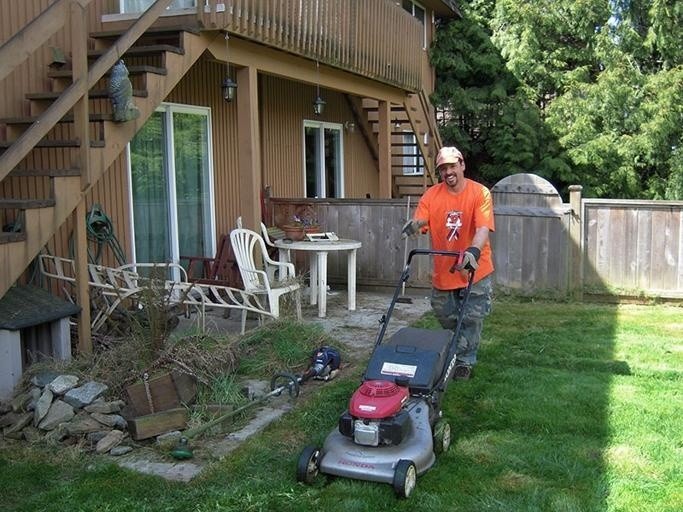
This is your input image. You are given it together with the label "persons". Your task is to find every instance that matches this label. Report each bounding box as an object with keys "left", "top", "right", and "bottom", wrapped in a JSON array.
[{"left": 400, "top": 146, "right": 496, "bottom": 381}]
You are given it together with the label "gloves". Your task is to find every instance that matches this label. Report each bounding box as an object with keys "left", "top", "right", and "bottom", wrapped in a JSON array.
[
  {"left": 449, "top": 247, "right": 481, "bottom": 274},
  {"left": 400, "top": 220, "right": 427, "bottom": 241}
]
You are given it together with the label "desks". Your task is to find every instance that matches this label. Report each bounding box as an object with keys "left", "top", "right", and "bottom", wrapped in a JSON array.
[{"left": 273, "top": 234, "right": 361, "bottom": 318}]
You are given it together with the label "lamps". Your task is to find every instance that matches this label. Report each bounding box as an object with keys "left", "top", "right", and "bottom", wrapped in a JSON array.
[
  {"left": 313, "top": 59, "right": 326, "bottom": 116},
  {"left": 220, "top": 31, "right": 239, "bottom": 103}
]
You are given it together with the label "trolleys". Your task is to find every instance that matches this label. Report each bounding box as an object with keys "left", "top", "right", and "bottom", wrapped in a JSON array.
[{"left": 296, "top": 249, "right": 475, "bottom": 498}]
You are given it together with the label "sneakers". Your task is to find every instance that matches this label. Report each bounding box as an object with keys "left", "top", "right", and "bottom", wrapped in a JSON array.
[{"left": 452, "top": 363, "right": 476, "bottom": 382}]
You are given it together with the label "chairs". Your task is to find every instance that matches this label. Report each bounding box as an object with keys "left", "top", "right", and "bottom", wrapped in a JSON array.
[{"left": 176, "top": 220, "right": 303, "bottom": 328}]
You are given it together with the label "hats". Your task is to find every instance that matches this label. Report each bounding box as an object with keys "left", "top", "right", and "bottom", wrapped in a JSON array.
[{"left": 435, "top": 146, "right": 464, "bottom": 170}]
